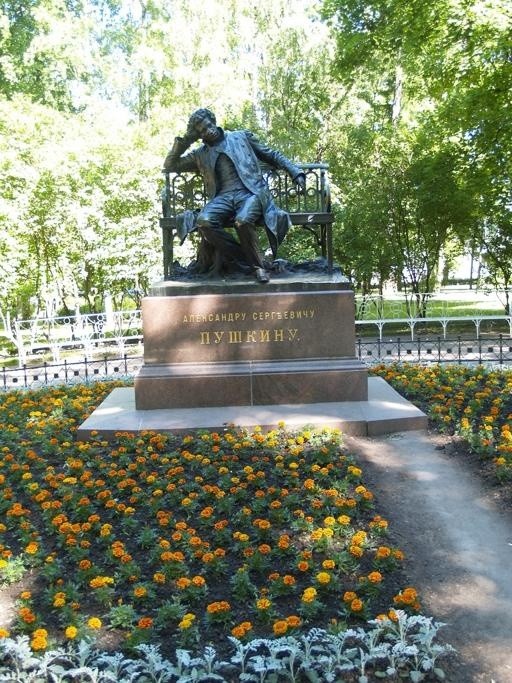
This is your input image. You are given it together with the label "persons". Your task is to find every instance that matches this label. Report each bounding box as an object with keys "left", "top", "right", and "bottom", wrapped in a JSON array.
[{"left": 164, "top": 109, "right": 306, "bottom": 282}]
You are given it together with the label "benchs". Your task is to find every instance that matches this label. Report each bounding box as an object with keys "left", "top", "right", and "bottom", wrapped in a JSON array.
[{"left": 161, "top": 163, "right": 334, "bottom": 277}]
